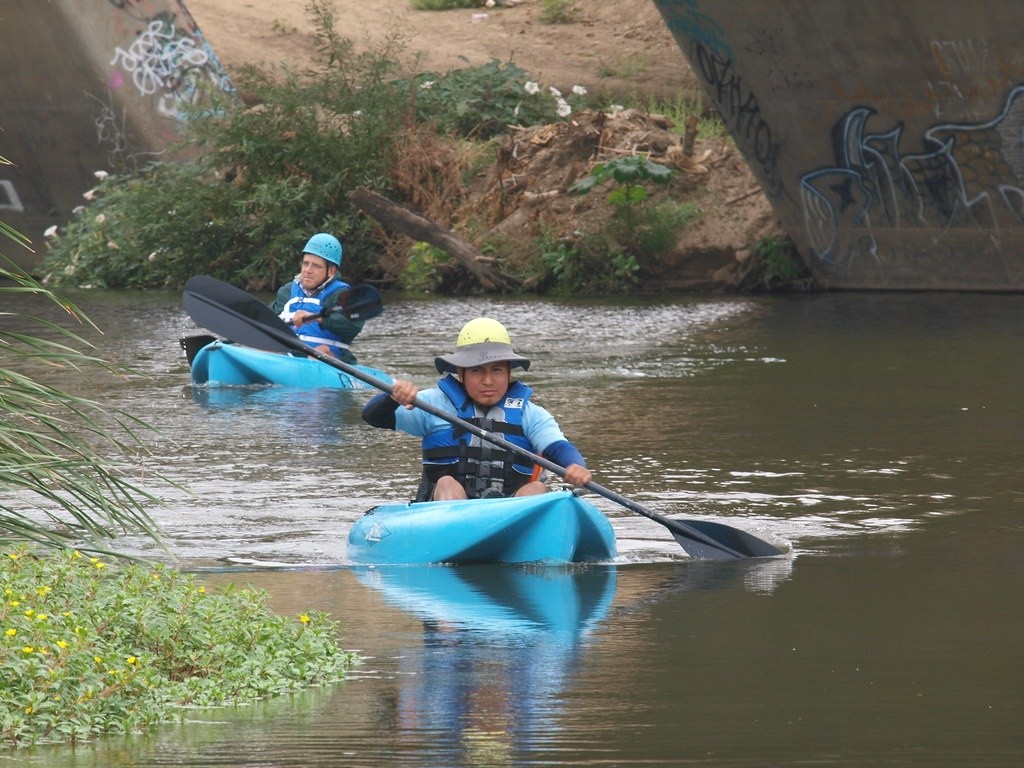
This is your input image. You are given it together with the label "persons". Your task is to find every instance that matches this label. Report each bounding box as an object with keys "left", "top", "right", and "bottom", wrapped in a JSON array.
[
  {"left": 361, "top": 317, "right": 591, "bottom": 503},
  {"left": 267, "top": 233, "right": 363, "bottom": 365}
]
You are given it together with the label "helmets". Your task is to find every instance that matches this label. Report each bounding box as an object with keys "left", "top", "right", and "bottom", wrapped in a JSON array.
[
  {"left": 432, "top": 315, "right": 531, "bottom": 375},
  {"left": 300, "top": 233, "right": 343, "bottom": 270}
]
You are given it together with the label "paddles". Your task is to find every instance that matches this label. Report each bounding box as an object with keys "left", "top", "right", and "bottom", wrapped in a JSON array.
[
  {"left": 179, "top": 285, "right": 383, "bottom": 367},
  {"left": 183, "top": 275, "right": 781, "bottom": 556}
]
